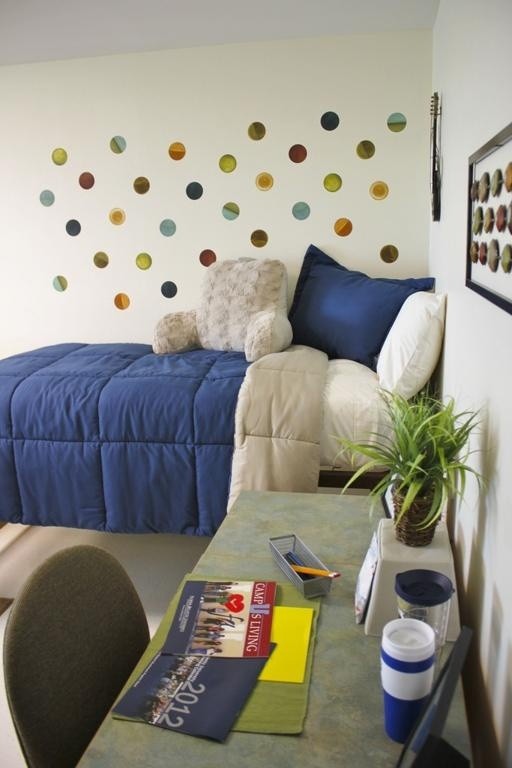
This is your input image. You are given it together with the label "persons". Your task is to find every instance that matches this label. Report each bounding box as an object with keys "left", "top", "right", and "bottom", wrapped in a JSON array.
[{"left": 139, "top": 581, "right": 238, "bottom": 723}]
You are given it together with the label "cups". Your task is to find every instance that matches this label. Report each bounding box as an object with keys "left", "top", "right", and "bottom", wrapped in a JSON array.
[{"left": 379, "top": 569, "right": 456, "bottom": 744}]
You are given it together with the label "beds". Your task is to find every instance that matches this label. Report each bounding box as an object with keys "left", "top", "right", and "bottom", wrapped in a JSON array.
[{"left": 1, "top": 342, "right": 436, "bottom": 489}]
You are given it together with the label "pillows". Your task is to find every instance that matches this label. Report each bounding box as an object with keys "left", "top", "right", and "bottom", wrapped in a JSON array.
[{"left": 288, "top": 244, "right": 447, "bottom": 400}]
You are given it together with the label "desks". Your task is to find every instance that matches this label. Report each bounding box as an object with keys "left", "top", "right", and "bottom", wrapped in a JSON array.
[{"left": 74, "top": 490, "right": 474, "bottom": 768}]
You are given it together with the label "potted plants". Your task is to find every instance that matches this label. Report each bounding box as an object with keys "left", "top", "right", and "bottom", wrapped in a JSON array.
[{"left": 325, "top": 386, "right": 492, "bottom": 547}]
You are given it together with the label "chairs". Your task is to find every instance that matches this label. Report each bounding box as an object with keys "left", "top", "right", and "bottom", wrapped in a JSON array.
[{"left": 2, "top": 545, "right": 149, "bottom": 768}]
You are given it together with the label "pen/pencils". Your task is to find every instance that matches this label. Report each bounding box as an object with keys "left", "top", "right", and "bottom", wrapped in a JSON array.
[{"left": 282, "top": 552, "right": 341, "bottom": 580}]
